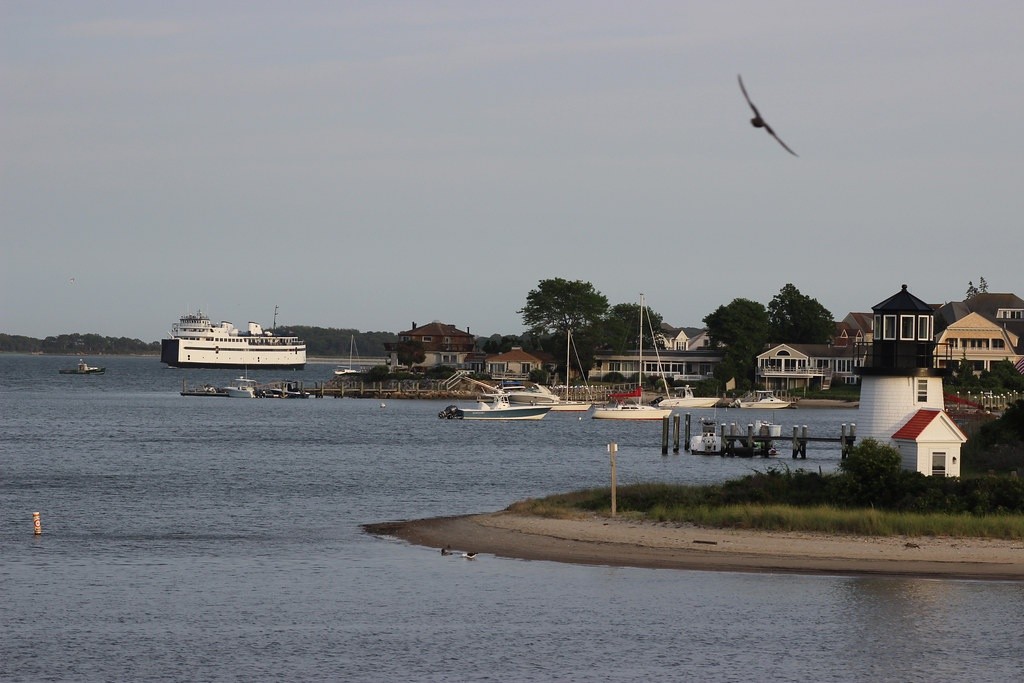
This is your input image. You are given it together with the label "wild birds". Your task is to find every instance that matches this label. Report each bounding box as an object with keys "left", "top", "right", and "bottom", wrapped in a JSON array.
[
  {"left": 734, "top": 74, "right": 798, "bottom": 158},
  {"left": 462, "top": 552, "right": 478, "bottom": 558}
]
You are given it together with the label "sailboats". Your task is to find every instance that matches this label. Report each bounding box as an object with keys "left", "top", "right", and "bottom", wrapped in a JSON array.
[
  {"left": 528, "top": 330, "right": 593, "bottom": 412},
  {"left": 333, "top": 334, "right": 367, "bottom": 376},
  {"left": 588, "top": 291, "right": 674, "bottom": 420}
]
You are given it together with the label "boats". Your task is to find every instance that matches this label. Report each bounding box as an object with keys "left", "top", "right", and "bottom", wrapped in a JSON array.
[
  {"left": 59, "top": 357, "right": 107, "bottom": 375},
  {"left": 475, "top": 380, "right": 562, "bottom": 405},
  {"left": 727, "top": 389, "right": 793, "bottom": 409},
  {"left": 159, "top": 304, "right": 307, "bottom": 369},
  {"left": 222, "top": 376, "right": 258, "bottom": 399},
  {"left": 437, "top": 391, "right": 554, "bottom": 421},
  {"left": 269, "top": 386, "right": 310, "bottom": 399},
  {"left": 657, "top": 384, "right": 721, "bottom": 407}
]
